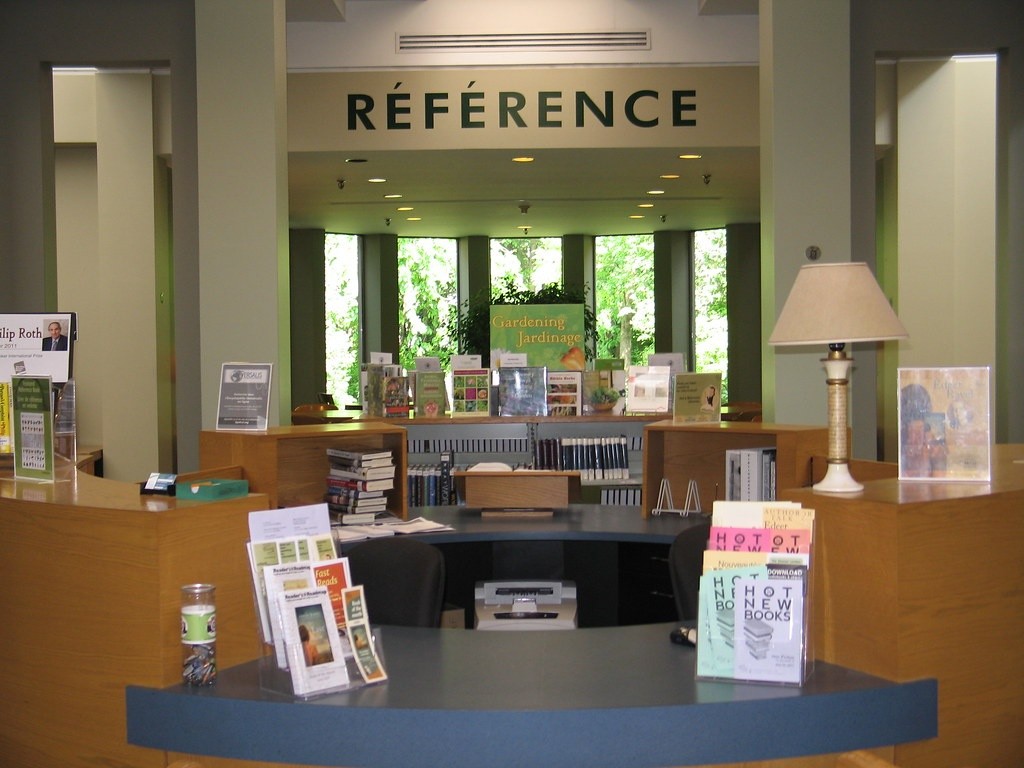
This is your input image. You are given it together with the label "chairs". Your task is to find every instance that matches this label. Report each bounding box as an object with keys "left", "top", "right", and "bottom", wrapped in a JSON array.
[
  {"left": 346, "top": 536, "right": 447, "bottom": 628},
  {"left": 669, "top": 523, "right": 711, "bottom": 620}
]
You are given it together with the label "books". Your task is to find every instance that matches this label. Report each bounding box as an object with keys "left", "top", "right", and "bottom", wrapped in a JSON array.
[{"left": 325, "top": 351, "right": 776, "bottom": 543}]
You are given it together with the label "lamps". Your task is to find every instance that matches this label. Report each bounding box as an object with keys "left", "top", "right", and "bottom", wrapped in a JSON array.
[{"left": 768, "top": 262, "right": 908, "bottom": 491}]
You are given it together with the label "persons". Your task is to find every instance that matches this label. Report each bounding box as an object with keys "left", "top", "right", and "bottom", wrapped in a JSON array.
[
  {"left": 298, "top": 624, "right": 362, "bottom": 667},
  {"left": 42, "top": 321, "right": 68, "bottom": 351}
]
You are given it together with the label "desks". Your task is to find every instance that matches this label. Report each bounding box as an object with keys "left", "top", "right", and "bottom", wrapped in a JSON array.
[{"left": 0, "top": 405, "right": 1024, "bottom": 768}]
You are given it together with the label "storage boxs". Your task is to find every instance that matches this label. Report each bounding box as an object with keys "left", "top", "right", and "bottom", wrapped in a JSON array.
[{"left": 175, "top": 479, "right": 248, "bottom": 501}]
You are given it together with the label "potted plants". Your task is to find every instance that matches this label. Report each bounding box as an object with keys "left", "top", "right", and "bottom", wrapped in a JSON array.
[{"left": 589, "top": 387, "right": 620, "bottom": 411}]
